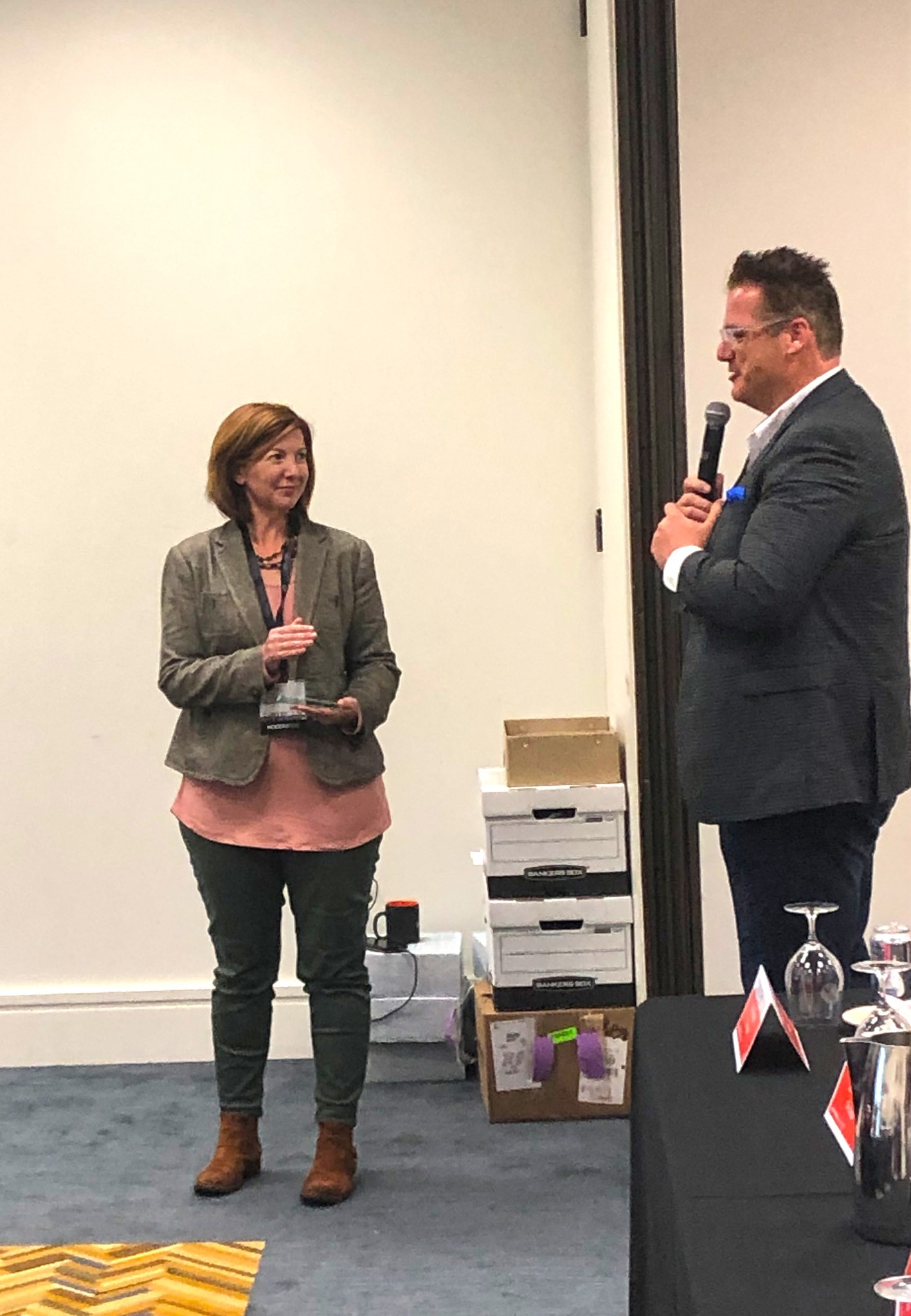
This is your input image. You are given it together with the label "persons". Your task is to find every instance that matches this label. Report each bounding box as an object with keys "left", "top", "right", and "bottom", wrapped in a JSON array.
[
  {"left": 651, "top": 243, "right": 909, "bottom": 998},
  {"left": 156, "top": 401, "right": 402, "bottom": 1207}
]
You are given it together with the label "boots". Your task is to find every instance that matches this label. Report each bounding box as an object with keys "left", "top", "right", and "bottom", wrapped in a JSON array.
[
  {"left": 192, "top": 1112, "right": 262, "bottom": 1195},
  {"left": 297, "top": 1124, "right": 358, "bottom": 1207}
]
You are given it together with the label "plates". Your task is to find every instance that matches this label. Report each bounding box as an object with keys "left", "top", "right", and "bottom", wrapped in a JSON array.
[{"left": 841, "top": 1004, "right": 876, "bottom": 1029}]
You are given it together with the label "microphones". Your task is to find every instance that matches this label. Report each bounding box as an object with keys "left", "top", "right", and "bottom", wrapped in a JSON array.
[{"left": 696, "top": 402, "right": 730, "bottom": 503}]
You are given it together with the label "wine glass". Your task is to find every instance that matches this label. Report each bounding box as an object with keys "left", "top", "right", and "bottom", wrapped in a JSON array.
[
  {"left": 783, "top": 900, "right": 845, "bottom": 1029},
  {"left": 850, "top": 960, "right": 911, "bottom": 1038},
  {"left": 872, "top": 1275, "right": 911, "bottom": 1316}
]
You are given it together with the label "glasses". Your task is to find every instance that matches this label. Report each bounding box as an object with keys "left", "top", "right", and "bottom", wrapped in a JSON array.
[{"left": 720, "top": 317, "right": 810, "bottom": 347}]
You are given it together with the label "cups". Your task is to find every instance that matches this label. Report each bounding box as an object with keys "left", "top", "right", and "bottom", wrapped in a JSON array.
[
  {"left": 372, "top": 901, "right": 420, "bottom": 943},
  {"left": 869, "top": 923, "right": 911, "bottom": 961}
]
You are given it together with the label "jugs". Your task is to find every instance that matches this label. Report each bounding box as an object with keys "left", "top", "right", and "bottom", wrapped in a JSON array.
[{"left": 838, "top": 1032, "right": 911, "bottom": 1247}]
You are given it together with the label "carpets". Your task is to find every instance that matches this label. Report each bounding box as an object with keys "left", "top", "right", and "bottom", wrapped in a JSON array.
[{"left": 0, "top": 1240, "right": 268, "bottom": 1316}]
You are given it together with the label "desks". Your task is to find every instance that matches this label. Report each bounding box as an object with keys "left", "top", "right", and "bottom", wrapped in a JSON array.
[{"left": 630, "top": 985, "right": 911, "bottom": 1316}]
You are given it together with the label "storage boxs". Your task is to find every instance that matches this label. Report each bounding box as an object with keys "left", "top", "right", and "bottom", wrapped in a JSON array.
[{"left": 362, "top": 714, "right": 638, "bottom": 1123}]
